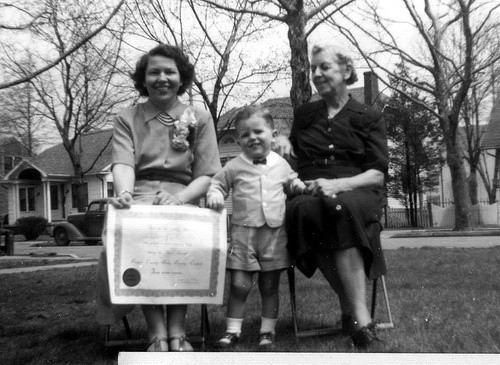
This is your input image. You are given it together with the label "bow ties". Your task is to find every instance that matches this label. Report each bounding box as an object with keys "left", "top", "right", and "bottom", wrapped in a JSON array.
[{"left": 253, "top": 158, "right": 266, "bottom": 165}]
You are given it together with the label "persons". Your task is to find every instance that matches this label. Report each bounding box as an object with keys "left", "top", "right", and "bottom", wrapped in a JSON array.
[
  {"left": 205, "top": 104, "right": 309, "bottom": 348},
  {"left": 269, "top": 43, "right": 389, "bottom": 350},
  {"left": 96, "top": 44, "right": 222, "bottom": 352}
]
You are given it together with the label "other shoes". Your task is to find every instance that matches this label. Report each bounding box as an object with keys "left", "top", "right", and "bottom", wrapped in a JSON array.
[
  {"left": 342, "top": 315, "right": 376, "bottom": 344},
  {"left": 215, "top": 331, "right": 242, "bottom": 347},
  {"left": 258, "top": 331, "right": 277, "bottom": 350}
]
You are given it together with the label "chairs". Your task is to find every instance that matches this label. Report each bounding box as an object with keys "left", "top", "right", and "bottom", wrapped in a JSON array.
[
  {"left": 103, "top": 194, "right": 211, "bottom": 353},
  {"left": 287, "top": 154, "right": 395, "bottom": 341}
]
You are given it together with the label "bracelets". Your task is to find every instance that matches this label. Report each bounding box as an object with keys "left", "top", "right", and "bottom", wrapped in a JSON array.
[{"left": 118, "top": 189, "right": 134, "bottom": 197}]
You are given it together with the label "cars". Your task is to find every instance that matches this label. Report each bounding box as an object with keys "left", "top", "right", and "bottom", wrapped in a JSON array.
[{"left": 49, "top": 198, "right": 111, "bottom": 246}]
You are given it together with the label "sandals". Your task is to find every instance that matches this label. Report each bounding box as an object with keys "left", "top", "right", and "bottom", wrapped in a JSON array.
[
  {"left": 169, "top": 335, "right": 195, "bottom": 352},
  {"left": 146, "top": 336, "right": 168, "bottom": 352}
]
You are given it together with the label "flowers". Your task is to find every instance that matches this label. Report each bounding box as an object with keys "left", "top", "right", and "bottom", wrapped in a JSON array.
[{"left": 171, "top": 108, "right": 197, "bottom": 152}]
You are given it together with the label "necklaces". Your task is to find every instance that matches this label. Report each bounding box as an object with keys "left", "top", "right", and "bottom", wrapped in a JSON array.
[{"left": 157, "top": 114, "right": 175, "bottom": 126}]
